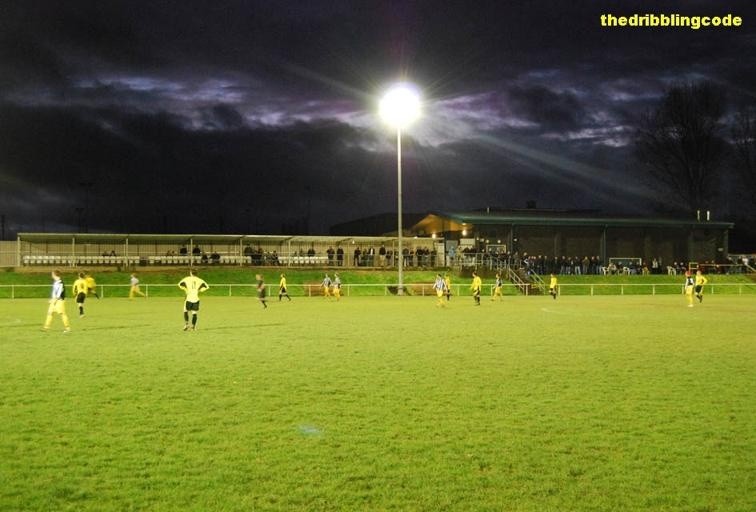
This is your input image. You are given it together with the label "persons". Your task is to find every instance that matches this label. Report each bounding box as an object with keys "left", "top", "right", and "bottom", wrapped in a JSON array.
[
  {"left": 469, "top": 270, "right": 482, "bottom": 306},
  {"left": 84, "top": 273, "right": 101, "bottom": 300},
  {"left": 104, "top": 239, "right": 756, "bottom": 301},
  {"left": 177, "top": 268, "right": 210, "bottom": 333},
  {"left": 253, "top": 272, "right": 268, "bottom": 309},
  {"left": 72, "top": 271, "right": 88, "bottom": 320},
  {"left": 40, "top": 269, "right": 71, "bottom": 335},
  {"left": 686, "top": 269, "right": 695, "bottom": 309},
  {"left": 432, "top": 274, "right": 448, "bottom": 310}
]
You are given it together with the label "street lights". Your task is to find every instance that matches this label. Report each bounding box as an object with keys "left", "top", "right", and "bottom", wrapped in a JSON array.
[{"left": 372, "top": 79, "right": 424, "bottom": 296}]
